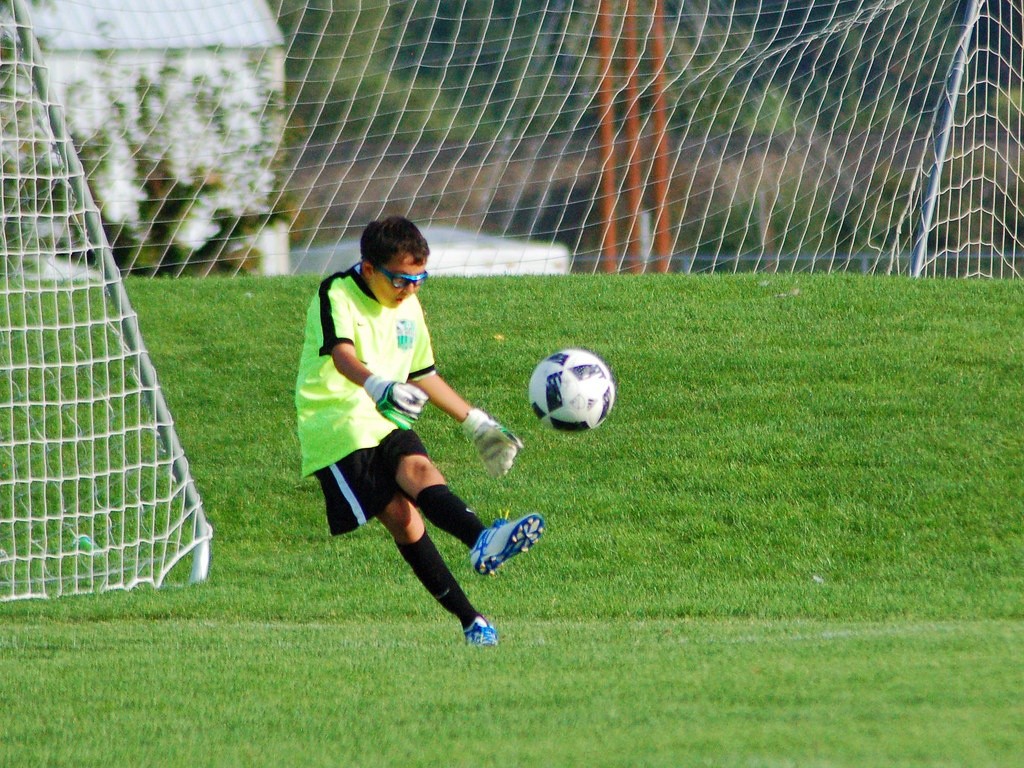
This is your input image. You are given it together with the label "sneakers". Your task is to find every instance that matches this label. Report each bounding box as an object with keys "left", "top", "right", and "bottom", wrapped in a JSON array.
[
  {"left": 464, "top": 614, "right": 497, "bottom": 647},
  {"left": 470, "top": 513, "right": 545, "bottom": 576}
]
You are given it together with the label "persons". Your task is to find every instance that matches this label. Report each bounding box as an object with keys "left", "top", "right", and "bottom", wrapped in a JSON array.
[{"left": 295, "top": 215, "right": 544, "bottom": 646}]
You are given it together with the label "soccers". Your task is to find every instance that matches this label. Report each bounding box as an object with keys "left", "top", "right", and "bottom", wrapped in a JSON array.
[{"left": 528, "top": 347, "right": 617, "bottom": 432}]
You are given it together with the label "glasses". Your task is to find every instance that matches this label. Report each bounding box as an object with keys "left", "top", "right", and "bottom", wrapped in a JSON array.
[{"left": 364, "top": 258, "right": 428, "bottom": 288}]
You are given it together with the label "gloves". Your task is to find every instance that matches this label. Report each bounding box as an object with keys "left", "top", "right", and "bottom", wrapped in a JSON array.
[
  {"left": 363, "top": 374, "right": 428, "bottom": 430},
  {"left": 462, "top": 407, "right": 524, "bottom": 476}
]
